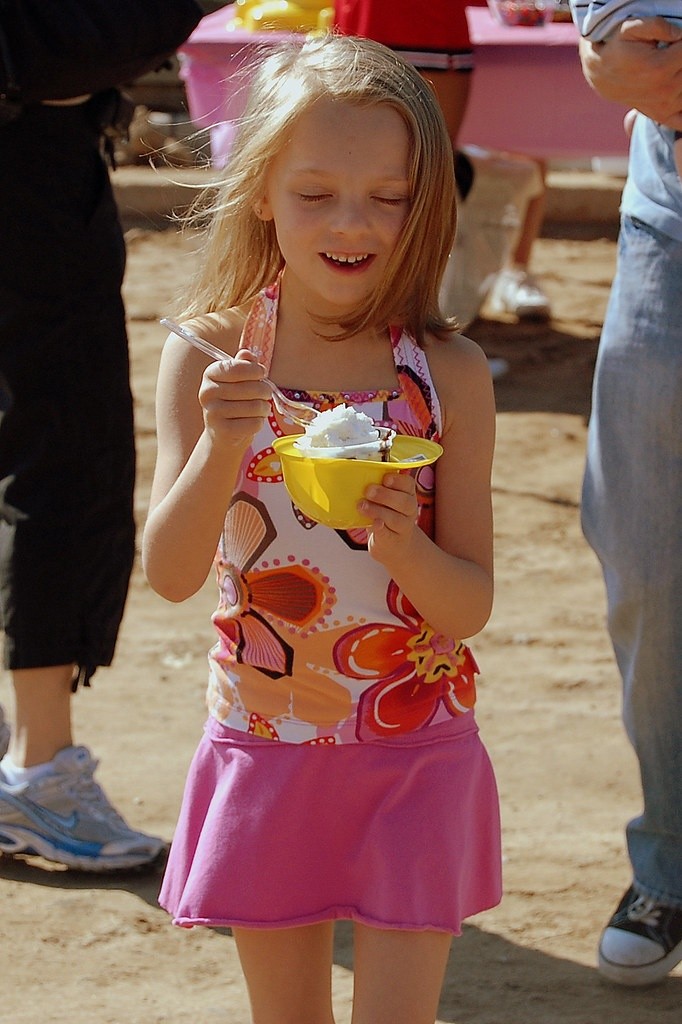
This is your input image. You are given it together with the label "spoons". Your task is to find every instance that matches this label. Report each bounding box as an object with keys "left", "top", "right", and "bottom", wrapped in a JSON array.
[{"left": 162, "top": 317, "right": 319, "bottom": 428}]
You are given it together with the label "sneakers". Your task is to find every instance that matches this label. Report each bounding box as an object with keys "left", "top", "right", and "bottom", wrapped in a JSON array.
[
  {"left": 0, "top": 746, "right": 168, "bottom": 878},
  {"left": 597, "top": 883, "right": 682, "bottom": 986},
  {"left": 494, "top": 269, "right": 549, "bottom": 321}
]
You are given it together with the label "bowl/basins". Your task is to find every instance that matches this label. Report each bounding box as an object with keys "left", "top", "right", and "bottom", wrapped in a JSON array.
[{"left": 275, "top": 433, "right": 444, "bottom": 529}]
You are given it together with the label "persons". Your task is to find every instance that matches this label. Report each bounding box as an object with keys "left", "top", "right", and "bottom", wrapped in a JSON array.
[
  {"left": 335, "top": 0, "right": 550, "bottom": 324},
  {"left": 0, "top": 0, "right": 243, "bottom": 878},
  {"left": 140, "top": 33, "right": 502, "bottom": 1024},
  {"left": 568, "top": 0, "right": 682, "bottom": 985}
]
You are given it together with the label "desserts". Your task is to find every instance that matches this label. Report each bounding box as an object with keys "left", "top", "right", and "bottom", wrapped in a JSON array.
[{"left": 292, "top": 402, "right": 397, "bottom": 464}]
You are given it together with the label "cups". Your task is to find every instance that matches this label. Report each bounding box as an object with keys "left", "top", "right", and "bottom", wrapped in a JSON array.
[{"left": 292, "top": 427, "right": 397, "bottom": 464}]
